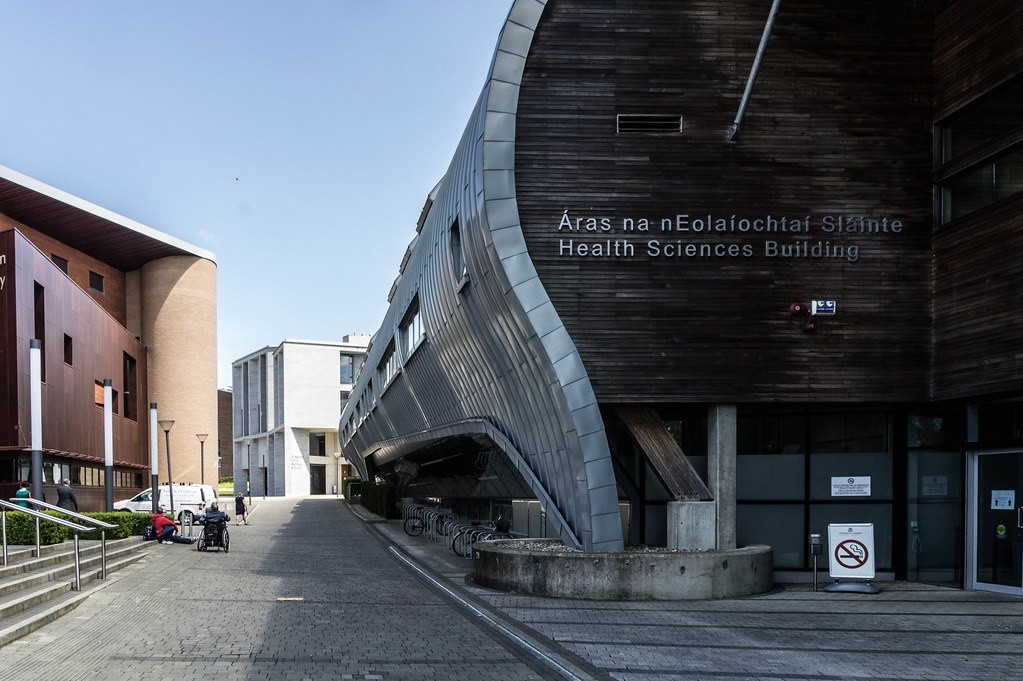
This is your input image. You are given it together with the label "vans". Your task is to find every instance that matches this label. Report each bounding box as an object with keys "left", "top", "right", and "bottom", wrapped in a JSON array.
[{"left": 113, "top": 482, "right": 219, "bottom": 526}]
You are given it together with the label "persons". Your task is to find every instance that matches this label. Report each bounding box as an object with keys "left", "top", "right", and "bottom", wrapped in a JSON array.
[
  {"left": 162, "top": 508, "right": 198, "bottom": 544},
  {"left": 57, "top": 478, "right": 80, "bottom": 512},
  {"left": 206, "top": 502, "right": 230, "bottom": 523},
  {"left": 151, "top": 507, "right": 179, "bottom": 544},
  {"left": 235, "top": 492, "right": 248, "bottom": 526},
  {"left": 16, "top": 481, "right": 32, "bottom": 508}
]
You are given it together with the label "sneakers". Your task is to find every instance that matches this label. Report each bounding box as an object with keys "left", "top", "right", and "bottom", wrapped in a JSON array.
[{"left": 162, "top": 540, "right": 173, "bottom": 544}]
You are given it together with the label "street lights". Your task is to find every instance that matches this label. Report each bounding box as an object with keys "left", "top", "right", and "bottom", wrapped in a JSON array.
[
  {"left": 243, "top": 438, "right": 253, "bottom": 505},
  {"left": 195, "top": 433, "right": 209, "bottom": 485},
  {"left": 158, "top": 419, "right": 176, "bottom": 522},
  {"left": 334, "top": 453, "right": 341, "bottom": 497}
]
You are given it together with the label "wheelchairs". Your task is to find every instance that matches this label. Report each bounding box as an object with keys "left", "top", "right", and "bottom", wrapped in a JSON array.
[{"left": 196, "top": 516, "right": 231, "bottom": 553}]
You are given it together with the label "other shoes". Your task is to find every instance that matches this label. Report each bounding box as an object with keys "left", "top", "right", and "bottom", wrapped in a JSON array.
[
  {"left": 245, "top": 522, "right": 249, "bottom": 525},
  {"left": 236, "top": 524, "right": 240, "bottom": 526}
]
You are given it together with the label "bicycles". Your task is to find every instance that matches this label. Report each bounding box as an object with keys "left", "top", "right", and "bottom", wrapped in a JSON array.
[
  {"left": 451, "top": 512, "right": 520, "bottom": 557},
  {"left": 403, "top": 503, "right": 456, "bottom": 537}
]
